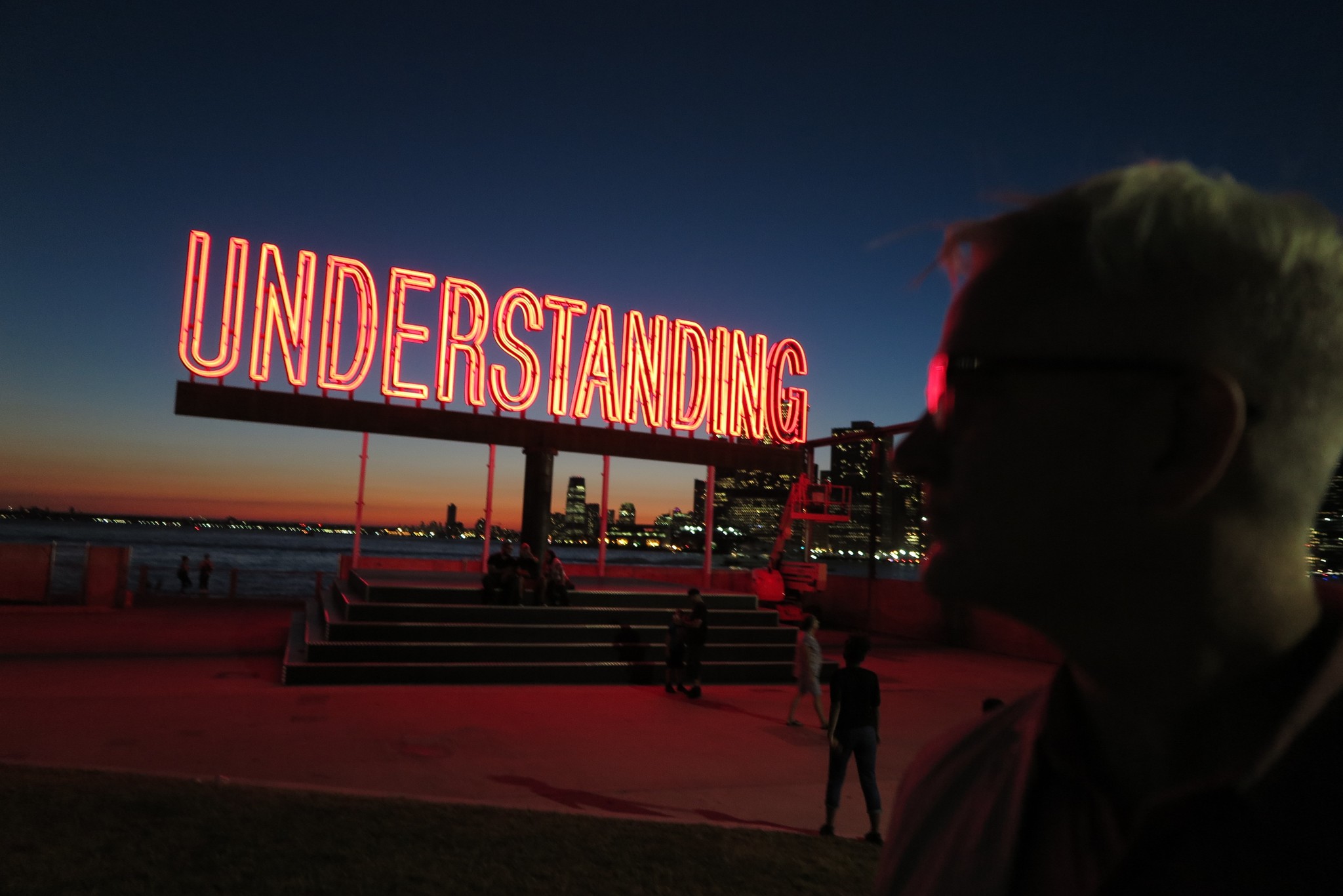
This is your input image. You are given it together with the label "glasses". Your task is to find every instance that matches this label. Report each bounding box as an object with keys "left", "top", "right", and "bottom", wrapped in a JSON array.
[{"left": 921, "top": 349, "right": 1138, "bottom": 416}]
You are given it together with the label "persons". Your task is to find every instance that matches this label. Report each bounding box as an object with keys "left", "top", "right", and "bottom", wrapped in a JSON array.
[
  {"left": 784, "top": 613, "right": 832, "bottom": 730},
  {"left": 872, "top": 159, "right": 1343, "bottom": 896},
  {"left": 229, "top": 567, "right": 240, "bottom": 603},
  {"left": 664, "top": 589, "right": 710, "bottom": 701},
  {"left": 175, "top": 555, "right": 193, "bottom": 596},
  {"left": 479, "top": 540, "right": 576, "bottom": 606},
  {"left": 816, "top": 636, "right": 884, "bottom": 845},
  {"left": 138, "top": 564, "right": 151, "bottom": 599},
  {"left": 199, "top": 553, "right": 214, "bottom": 601},
  {"left": 980, "top": 698, "right": 1006, "bottom": 713}
]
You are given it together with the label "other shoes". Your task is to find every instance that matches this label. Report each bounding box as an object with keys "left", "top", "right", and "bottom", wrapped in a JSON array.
[
  {"left": 820, "top": 826, "right": 834, "bottom": 835},
  {"left": 866, "top": 832, "right": 882, "bottom": 843}
]
